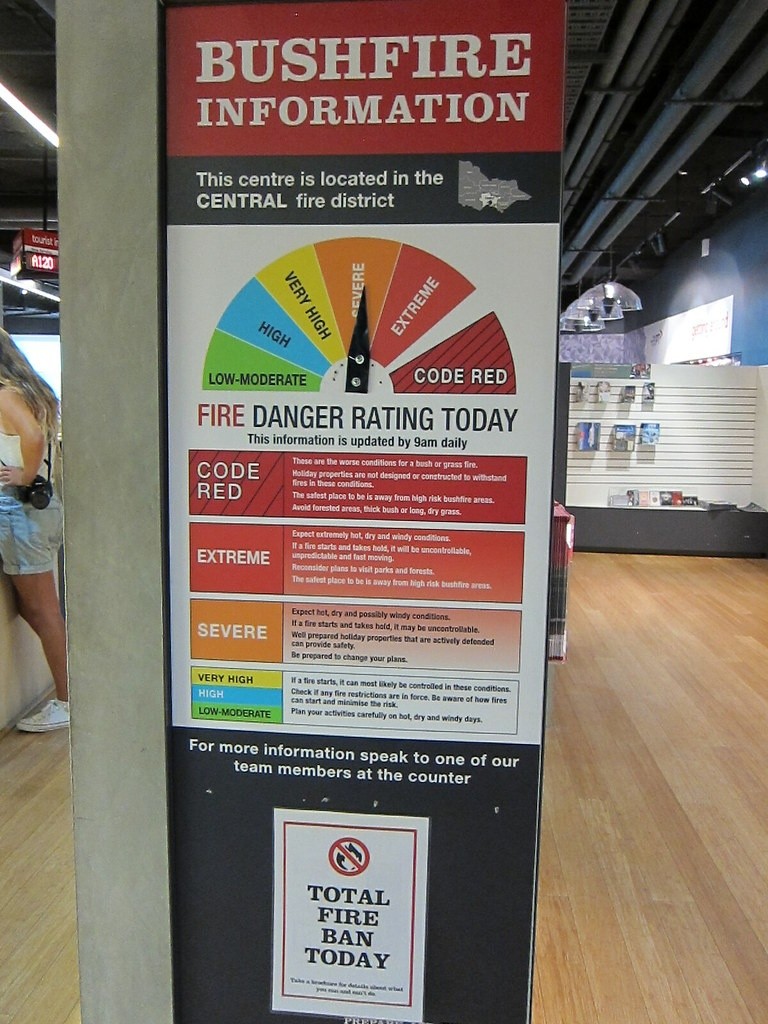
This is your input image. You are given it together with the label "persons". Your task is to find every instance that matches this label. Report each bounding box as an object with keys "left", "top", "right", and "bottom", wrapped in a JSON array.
[{"left": 0, "top": 328, "right": 71, "bottom": 733}]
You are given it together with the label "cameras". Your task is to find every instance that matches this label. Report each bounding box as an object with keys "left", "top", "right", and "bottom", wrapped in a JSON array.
[{"left": 17, "top": 474, "right": 54, "bottom": 509}]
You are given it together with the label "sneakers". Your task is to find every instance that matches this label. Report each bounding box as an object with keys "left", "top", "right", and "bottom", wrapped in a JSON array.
[{"left": 16, "top": 697, "right": 69, "bottom": 732}]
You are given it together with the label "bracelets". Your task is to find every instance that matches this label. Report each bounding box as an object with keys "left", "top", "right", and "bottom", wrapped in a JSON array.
[{"left": 20, "top": 472, "right": 31, "bottom": 488}]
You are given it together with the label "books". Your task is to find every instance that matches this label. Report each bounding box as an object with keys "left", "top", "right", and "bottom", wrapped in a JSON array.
[
  {"left": 700, "top": 499, "right": 737, "bottom": 510},
  {"left": 683, "top": 496, "right": 698, "bottom": 506},
  {"left": 627, "top": 490, "right": 684, "bottom": 507}
]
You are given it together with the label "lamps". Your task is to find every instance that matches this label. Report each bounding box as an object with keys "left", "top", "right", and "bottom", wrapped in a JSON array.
[
  {"left": 647, "top": 224, "right": 668, "bottom": 256},
  {"left": 559, "top": 307, "right": 608, "bottom": 335},
  {"left": 0, "top": 79, "right": 59, "bottom": 150},
  {"left": 0, "top": 262, "right": 60, "bottom": 302},
  {"left": 703, "top": 150, "right": 768, "bottom": 217},
  {"left": 578, "top": 242, "right": 643, "bottom": 312},
  {"left": 565, "top": 278, "right": 628, "bottom": 322}
]
